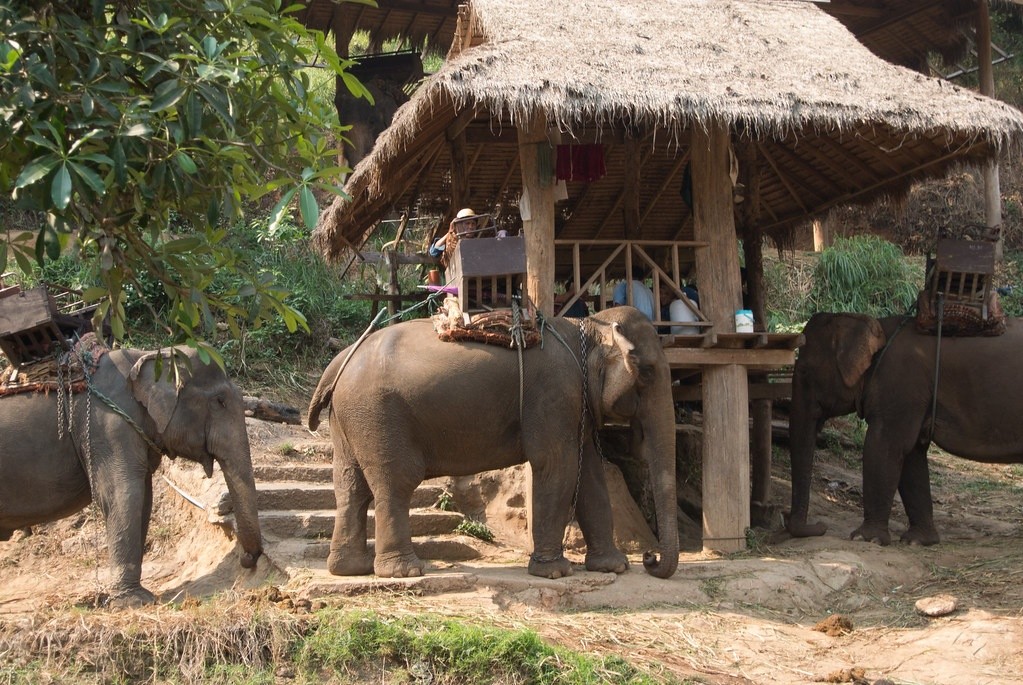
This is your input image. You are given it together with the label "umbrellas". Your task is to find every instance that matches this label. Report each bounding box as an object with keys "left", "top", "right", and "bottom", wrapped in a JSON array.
[{"left": 418, "top": 286, "right": 506, "bottom": 299}]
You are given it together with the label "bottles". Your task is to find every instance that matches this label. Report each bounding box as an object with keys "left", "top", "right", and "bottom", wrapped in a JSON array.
[{"left": 669, "top": 293, "right": 699, "bottom": 334}]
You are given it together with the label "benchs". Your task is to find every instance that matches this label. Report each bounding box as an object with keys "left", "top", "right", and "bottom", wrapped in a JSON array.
[
  {"left": 924, "top": 222, "right": 1001, "bottom": 327},
  {"left": 0, "top": 272, "right": 112, "bottom": 371},
  {"left": 442, "top": 236, "right": 524, "bottom": 309}
]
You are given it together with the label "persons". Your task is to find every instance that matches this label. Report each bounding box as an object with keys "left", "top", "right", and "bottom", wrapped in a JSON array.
[
  {"left": 430, "top": 208, "right": 518, "bottom": 305},
  {"left": 740, "top": 267, "right": 763, "bottom": 324},
  {"left": 555, "top": 276, "right": 589, "bottom": 318},
  {"left": 649, "top": 270, "right": 700, "bottom": 335},
  {"left": 614, "top": 265, "right": 658, "bottom": 331}
]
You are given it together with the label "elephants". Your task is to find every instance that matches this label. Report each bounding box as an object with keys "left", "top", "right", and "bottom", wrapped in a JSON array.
[
  {"left": 308, "top": 304, "right": 680, "bottom": 580},
  {"left": 782, "top": 311, "right": 1023, "bottom": 546},
  {"left": 0, "top": 340, "right": 263, "bottom": 613}
]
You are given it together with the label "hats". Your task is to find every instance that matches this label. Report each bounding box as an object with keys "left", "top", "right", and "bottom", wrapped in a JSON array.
[{"left": 451, "top": 208, "right": 490, "bottom": 226}]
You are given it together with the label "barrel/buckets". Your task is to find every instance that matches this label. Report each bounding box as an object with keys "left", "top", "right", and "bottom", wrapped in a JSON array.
[{"left": 735, "top": 309, "right": 754, "bottom": 333}]
